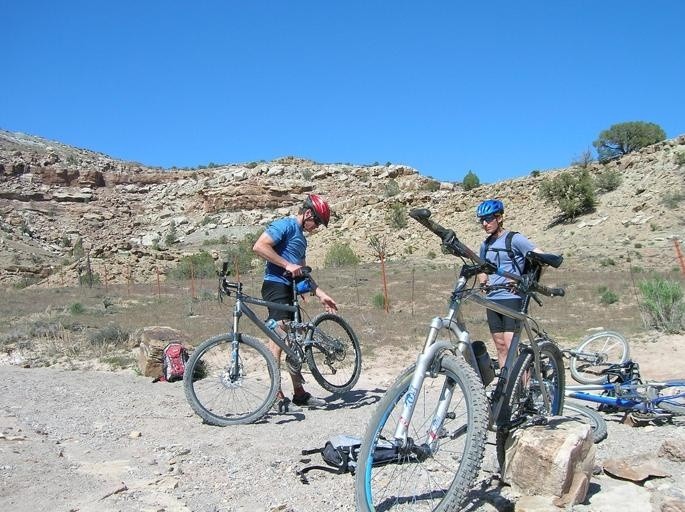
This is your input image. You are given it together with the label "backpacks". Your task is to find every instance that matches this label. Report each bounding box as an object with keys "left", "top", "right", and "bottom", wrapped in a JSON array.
[
  {"left": 483, "top": 231, "right": 542, "bottom": 290},
  {"left": 163, "top": 342, "right": 190, "bottom": 383}
]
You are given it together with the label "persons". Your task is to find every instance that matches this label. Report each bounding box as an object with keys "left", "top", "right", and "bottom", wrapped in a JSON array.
[
  {"left": 477, "top": 199, "right": 544, "bottom": 387},
  {"left": 253, "top": 194, "right": 338, "bottom": 412}
]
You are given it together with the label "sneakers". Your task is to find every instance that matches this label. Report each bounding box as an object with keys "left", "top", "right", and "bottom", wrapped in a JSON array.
[
  {"left": 272, "top": 396, "right": 302, "bottom": 412},
  {"left": 292, "top": 390, "right": 328, "bottom": 407}
]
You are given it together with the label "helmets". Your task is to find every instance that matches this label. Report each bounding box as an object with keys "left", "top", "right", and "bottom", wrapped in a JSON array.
[
  {"left": 304, "top": 191, "right": 330, "bottom": 228},
  {"left": 475, "top": 199, "right": 505, "bottom": 217}
]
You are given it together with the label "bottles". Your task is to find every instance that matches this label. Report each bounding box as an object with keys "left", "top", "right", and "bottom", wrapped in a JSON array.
[
  {"left": 265, "top": 318, "right": 288, "bottom": 341},
  {"left": 471, "top": 341, "right": 496, "bottom": 383}
]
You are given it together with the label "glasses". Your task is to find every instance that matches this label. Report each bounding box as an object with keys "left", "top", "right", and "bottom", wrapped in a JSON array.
[
  {"left": 314, "top": 218, "right": 322, "bottom": 227},
  {"left": 478, "top": 214, "right": 501, "bottom": 224}
]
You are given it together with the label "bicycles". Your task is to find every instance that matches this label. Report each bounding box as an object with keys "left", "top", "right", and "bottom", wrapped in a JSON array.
[{"left": 183, "top": 262, "right": 361, "bottom": 427}]
